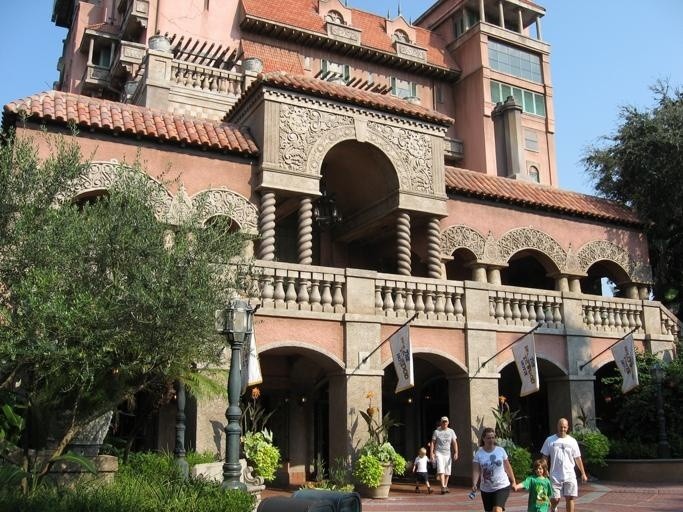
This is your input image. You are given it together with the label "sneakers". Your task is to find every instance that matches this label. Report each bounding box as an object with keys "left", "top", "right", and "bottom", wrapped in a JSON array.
[
  {"left": 416, "top": 489, "right": 420, "bottom": 493},
  {"left": 441, "top": 487, "right": 450, "bottom": 495},
  {"left": 428, "top": 489, "right": 433, "bottom": 494}
]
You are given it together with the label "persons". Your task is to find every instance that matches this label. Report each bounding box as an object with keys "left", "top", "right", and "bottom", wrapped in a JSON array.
[
  {"left": 430, "top": 416, "right": 458, "bottom": 493},
  {"left": 515, "top": 459, "right": 553, "bottom": 512},
  {"left": 413, "top": 448, "right": 434, "bottom": 494},
  {"left": 540, "top": 418, "right": 588, "bottom": 512},
  {"left": 427, "top": 422, "right": 441, "bottom": 480},
  {"left": 472, "top": 428, "right": 517, "bottom": 512}
]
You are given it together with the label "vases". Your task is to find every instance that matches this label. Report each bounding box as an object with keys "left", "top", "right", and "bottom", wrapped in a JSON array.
[{"left": 365, "top": 459, "right": 393, "bottom": 499}]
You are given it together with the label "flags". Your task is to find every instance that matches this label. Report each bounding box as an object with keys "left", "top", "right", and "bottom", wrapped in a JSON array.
[
  {"left": 511, "top": 334, "right": 540, "bottom": 397},
  {"left": 241, "top": 319, "right": 264, "bottom": 393},
  {"left": 388, "top": 323, "right": 415, "bottom": 393},
  {"left": 611, "top": 334, "right": 639, "bottom": 394}
]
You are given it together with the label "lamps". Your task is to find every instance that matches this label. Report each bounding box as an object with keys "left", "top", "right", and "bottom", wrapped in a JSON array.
[{"left": 314, "top": 163, "right": 344, "bottom": 231}]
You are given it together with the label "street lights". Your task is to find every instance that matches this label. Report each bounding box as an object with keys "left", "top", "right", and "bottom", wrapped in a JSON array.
[{"left": 214, "top": 301, "right": 254, "bottom": 491}]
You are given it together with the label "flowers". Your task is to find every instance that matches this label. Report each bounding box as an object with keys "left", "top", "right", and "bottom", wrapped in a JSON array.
[
  {"left": 349, "top": 390, "right": 406, "bottom": 488},
  {"left": 476, "top": 393, "right": 530, "bottom": 479},
  {"left": 240, "top": 389, "right": 283, "bottom": 482}
]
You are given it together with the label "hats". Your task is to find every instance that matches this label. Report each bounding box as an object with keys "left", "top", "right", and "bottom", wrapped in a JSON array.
[{"left": 441, "top": 416, "right": 448, "bottom": 422}]
